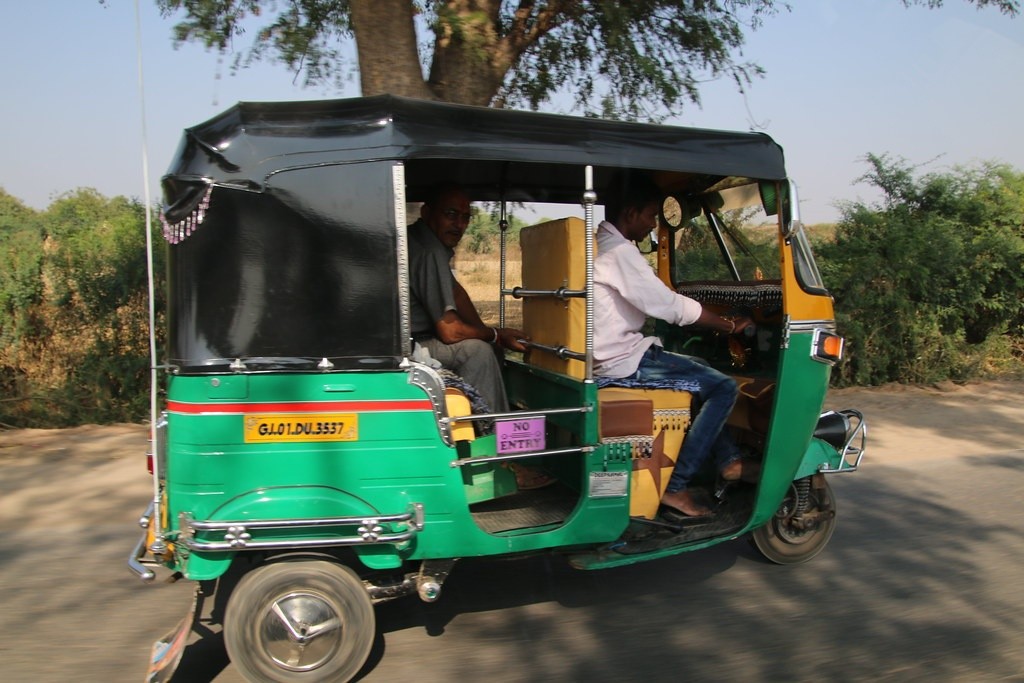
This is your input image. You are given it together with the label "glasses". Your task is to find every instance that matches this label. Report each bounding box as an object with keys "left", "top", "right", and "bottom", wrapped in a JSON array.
[{"left": 428, "top": 204, "right": 474, "bottom": 222}]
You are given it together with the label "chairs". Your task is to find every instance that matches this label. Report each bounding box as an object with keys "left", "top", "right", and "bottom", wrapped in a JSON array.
[{"left": 519, "top": 217, "right": 692, "bottom": 523}]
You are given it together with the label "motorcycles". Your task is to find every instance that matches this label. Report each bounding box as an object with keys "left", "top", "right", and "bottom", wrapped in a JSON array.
[{"left": 125, "top": 91, "right": 870, "bottom": 683}]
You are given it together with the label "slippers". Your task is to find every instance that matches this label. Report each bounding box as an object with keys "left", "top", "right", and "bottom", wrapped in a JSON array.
[{"left": 515, "top": 468, "right": 558, "bottom": 490}]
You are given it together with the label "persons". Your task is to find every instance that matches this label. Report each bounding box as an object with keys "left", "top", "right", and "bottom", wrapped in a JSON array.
[
  {"left": 406, "top": 180, "right": 558, "bottom": 490},
  {"left": 593, "top": 171, "right": 754, "bottom": 517}
]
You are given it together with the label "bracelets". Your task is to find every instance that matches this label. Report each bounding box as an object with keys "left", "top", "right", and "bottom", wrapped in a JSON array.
[
  {"left": 488, "top": 326, "right": 498, "bottom": 344},
  {"left": 719, "top": 314, "right": 736, "bottom": 334}
]
